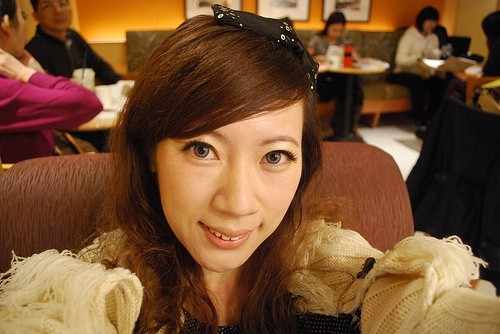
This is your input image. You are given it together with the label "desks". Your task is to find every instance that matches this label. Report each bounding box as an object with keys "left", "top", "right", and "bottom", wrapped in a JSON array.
[
  {"left": 73, "top": 83, "right": 129, "bottom": 130},
  {"left": 322, "top": 57, "right": 390, "bottom": 140}
]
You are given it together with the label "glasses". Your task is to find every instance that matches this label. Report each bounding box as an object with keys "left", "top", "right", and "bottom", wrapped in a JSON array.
[
  {"left": 39, "top": 1, "right": 71, "bottom": 12},
  {"left": 21, "top": 10, "right": 28, "bottom": 20}
]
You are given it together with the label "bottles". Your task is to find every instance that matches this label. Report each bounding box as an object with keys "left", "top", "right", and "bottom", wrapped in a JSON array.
[{"left": 343, "top": 38, "right": 354, "bottom": 68}]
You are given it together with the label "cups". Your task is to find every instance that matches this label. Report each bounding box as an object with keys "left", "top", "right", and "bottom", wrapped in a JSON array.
[{"left": 73, "top": 68, "right": 96, "bottom": 92}]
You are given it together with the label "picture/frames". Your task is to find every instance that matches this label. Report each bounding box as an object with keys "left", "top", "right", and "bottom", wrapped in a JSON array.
[
  {"left": 256, "top": 0, "right": 311, "bottom": 22},
  {"left": 184, "top": 0, "right": 243, "bottom": 19},
  {"left": 322, "top": 0, "right": 373, "bottom": 23}
]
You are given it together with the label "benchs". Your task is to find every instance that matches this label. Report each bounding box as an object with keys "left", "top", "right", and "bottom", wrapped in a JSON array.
[{"left": 127, "top": 30, "right": 411, "bottom": 128}]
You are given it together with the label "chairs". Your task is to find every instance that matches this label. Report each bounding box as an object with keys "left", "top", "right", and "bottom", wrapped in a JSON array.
[{"left": 0, "top": 140, "right": 414, "bottom": 271}]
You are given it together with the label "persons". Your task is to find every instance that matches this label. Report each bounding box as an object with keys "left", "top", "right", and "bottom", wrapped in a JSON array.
[
  {"left": 0, "top": 0, "right": 500, "bottom": 164},
  {"left": 0, "top": 3, "right": 499, "bottom": 334}
]
